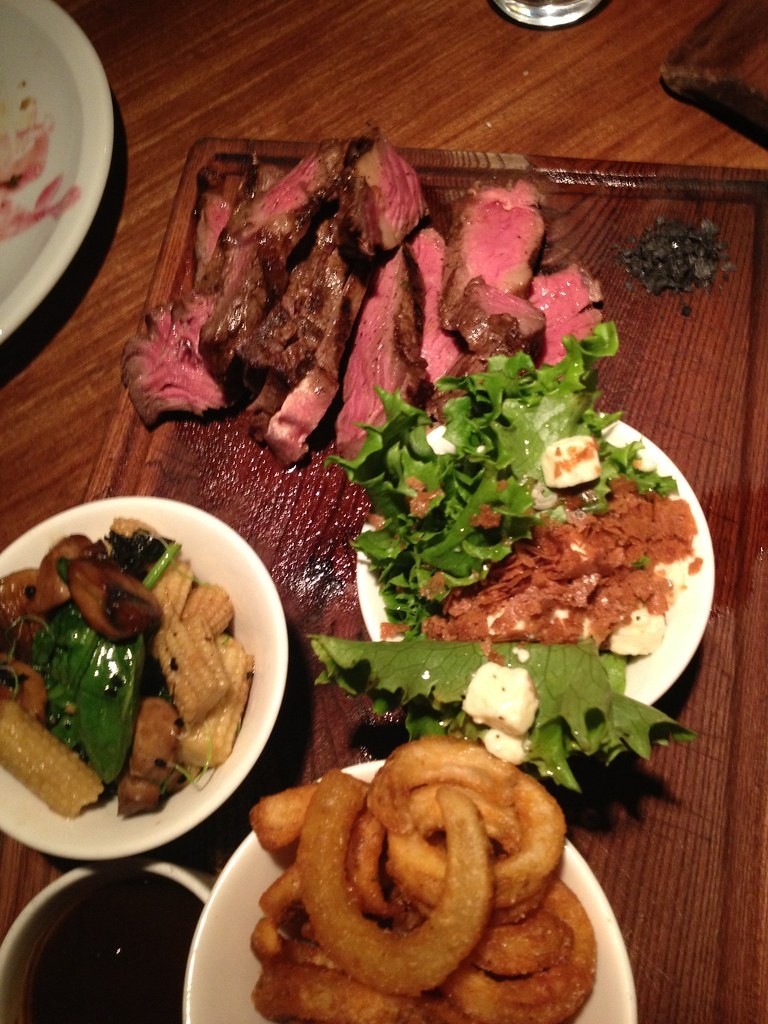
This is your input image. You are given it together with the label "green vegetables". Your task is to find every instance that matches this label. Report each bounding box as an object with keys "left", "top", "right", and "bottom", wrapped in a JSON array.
[{"left": 31, "top": 604, "right": 148, "bottom": 785}]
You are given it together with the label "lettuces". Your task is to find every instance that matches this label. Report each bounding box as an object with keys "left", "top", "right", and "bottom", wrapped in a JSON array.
[{"left": 312, "top": 324, "right": 702, "bottom": 790}]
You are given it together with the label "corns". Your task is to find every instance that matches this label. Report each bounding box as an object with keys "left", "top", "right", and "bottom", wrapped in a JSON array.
[
  {"left": 146, "top": 561, "right": 250, "bottom": 769},
  {"left": 0, "top": 698, "right": 104, "bottom": 818}
]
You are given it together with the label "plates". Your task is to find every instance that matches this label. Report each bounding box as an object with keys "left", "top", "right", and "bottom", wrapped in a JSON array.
[
  {"left": 183, "top": 759, "right": 637, "bottom": 1024},
  {"left": 0, "top": 0, "right": 114, "bottom": 344},
  {"left": 355, "top": 411, "right": 715, "bottom": 706},
  {"left": 0, "top": 495, "right": 289, "bottom": 862}
]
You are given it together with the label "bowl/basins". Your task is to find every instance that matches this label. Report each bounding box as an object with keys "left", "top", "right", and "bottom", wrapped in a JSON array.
[{"left": 0, "top": 857, "right": 215, "bottom": 1024}]
